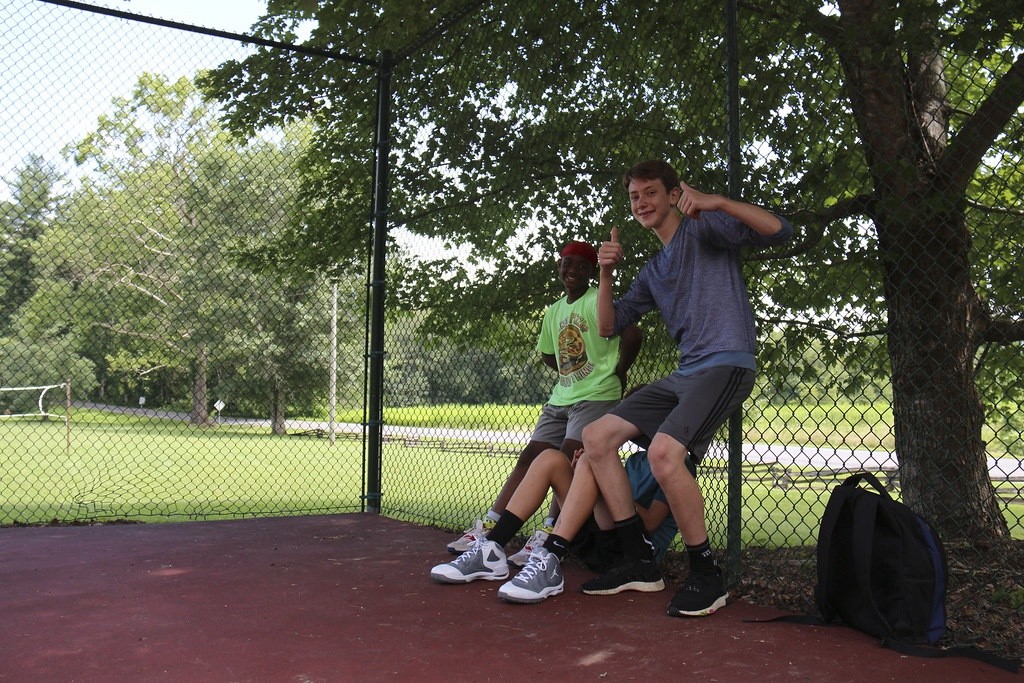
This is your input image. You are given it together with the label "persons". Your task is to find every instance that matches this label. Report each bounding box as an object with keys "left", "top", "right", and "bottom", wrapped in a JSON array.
[
  {"left": 446, "top": 241, "right": 643, "bottom": 568},
  {"left": 429, "top": 383, "right": 698, "bottom": 605},
  {"left": 580, "top": 160, "right": 795, "bottom": 619}
]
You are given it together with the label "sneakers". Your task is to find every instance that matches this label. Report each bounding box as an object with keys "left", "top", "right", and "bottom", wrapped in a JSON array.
[
  {"left": 581, "top": 553, "right": 665, "bottom": 595},
  {"left": 507, "top": 530, "right": 552, "bottom": 568},
  {"left": 498, "top": 545, "right": 565, "bottom": 603},
  {"left": 430, "top": 534, "right": 510, "bottom": 584},
  {"left": 666, "top": 565, "right": 726, "bottom": 617},
  {"left": 447, "top": 519, "right": 492, "bottom": 555}
]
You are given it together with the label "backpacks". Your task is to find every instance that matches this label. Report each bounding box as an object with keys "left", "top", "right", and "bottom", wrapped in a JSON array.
[{"left": 815, "top": 472, "right": 947, "bottom": 646}]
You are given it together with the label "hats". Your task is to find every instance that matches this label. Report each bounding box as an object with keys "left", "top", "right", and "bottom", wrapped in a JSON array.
[{"left": 561, "top": 242, "right": 599, "bottom": 268}]
